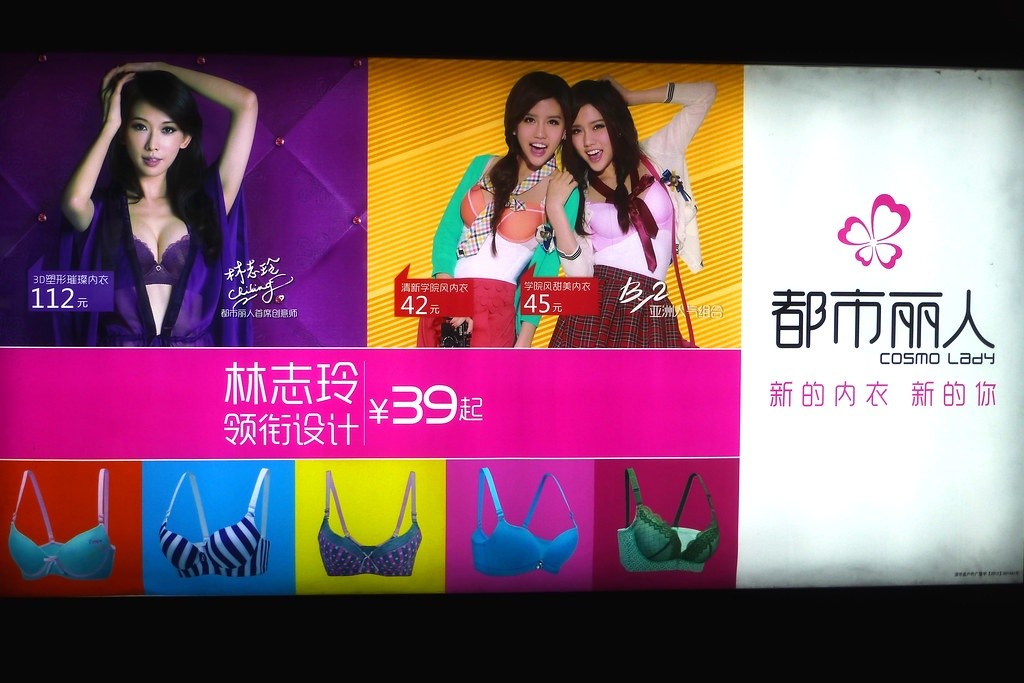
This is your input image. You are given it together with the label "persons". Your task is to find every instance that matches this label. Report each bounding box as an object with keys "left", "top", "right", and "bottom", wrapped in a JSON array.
[
  {"left": 54, "top": 62, "right": 257, "bottom": 346},
  {"left": 416, "top": 71, "right": 716, "bottom": 348}
]
